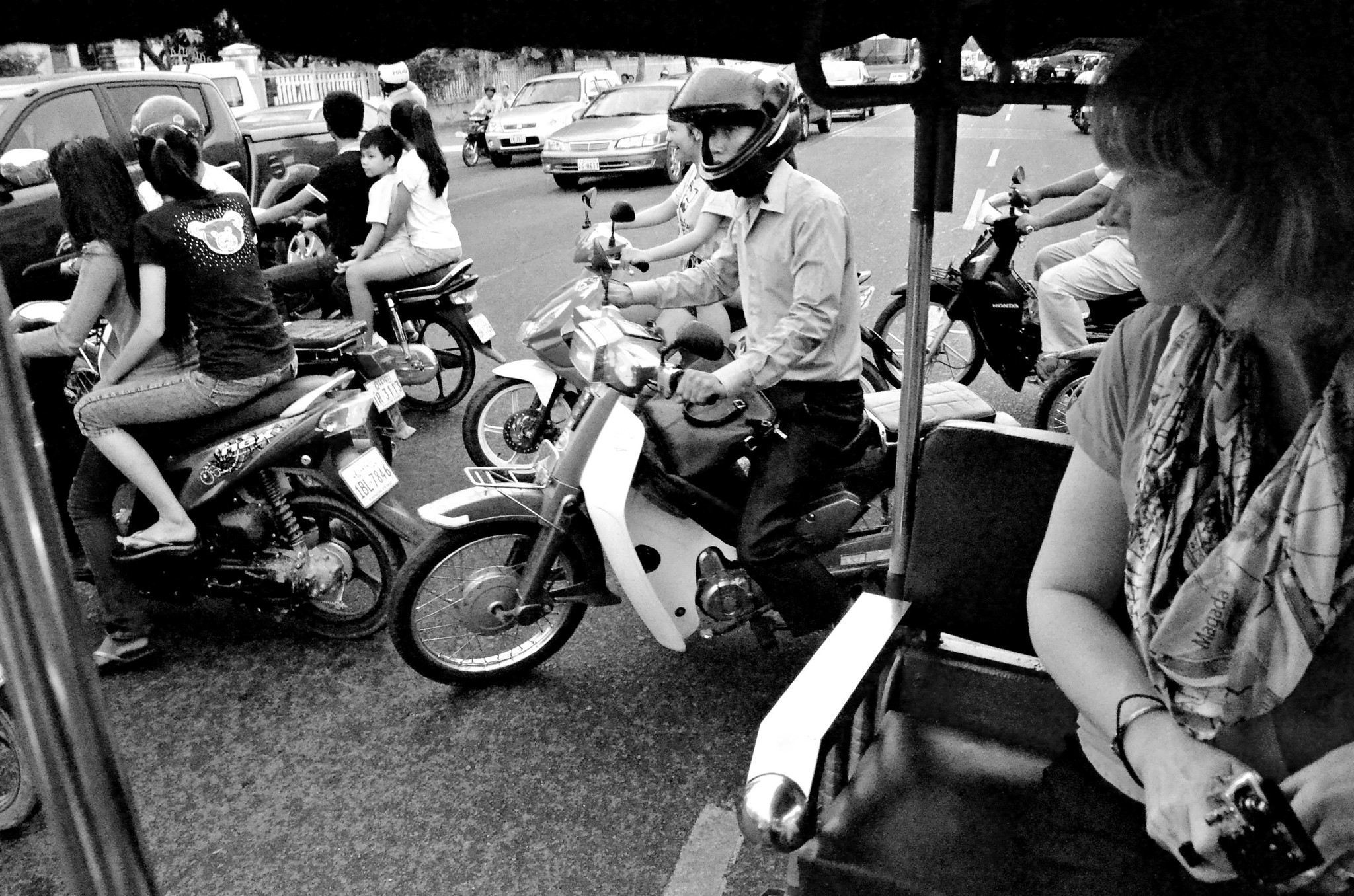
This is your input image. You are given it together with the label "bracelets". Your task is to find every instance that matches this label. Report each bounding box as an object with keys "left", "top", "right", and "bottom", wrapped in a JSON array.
[
  {"left": 1111, "top": 705, "right": 1168, "bottom": 756},
  {"left": 1115, "top": 692, "right": 1171, "bottom": 788}
]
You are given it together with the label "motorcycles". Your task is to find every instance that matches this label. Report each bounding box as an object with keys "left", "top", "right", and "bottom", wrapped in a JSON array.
[
  {"left": 1039, "top": 342, "right": 1100, "bottom": 436},
  {"left": 873, "top": 165, "right": 1148, "bottom": 392},
  {"left": 260, "top": 216, "right": 507, "bottom": 411},
  {"left": 459, "top": 193, "right": 913, "bottom": 481},
  {"left": 387, "top": 242, "right": 1035, "bottom": 690},
  {"left": 1070, "top": 104, "right": 1094, "bottom": 134},
  {"left": 461, "top": 110, "right": 490, "bottom": 166},
  {"left": 280, "top": 314, "right": 415, "bottom": 470}
]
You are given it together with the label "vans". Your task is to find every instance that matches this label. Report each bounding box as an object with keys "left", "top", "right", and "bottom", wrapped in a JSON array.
[{"left": 174, "top": 63, "right": 261, "bottom": 119}]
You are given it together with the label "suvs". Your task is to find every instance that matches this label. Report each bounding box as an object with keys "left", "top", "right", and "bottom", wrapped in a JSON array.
[{"left": 485, "top": 65, "right": 623, "bottom": 167}]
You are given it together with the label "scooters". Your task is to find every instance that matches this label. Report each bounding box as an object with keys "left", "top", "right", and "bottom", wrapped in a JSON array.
[{"left": 11, "top": 295, "right": 430, "bottom": 639}]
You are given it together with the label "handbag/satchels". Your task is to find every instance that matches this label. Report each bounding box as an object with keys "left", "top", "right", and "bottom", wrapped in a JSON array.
[{"left": 644, "top": 342, "right": 787, "bottom": 476}]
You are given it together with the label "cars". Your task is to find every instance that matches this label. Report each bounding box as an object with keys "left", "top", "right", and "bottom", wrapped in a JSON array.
[
  {"left": 822, "top": 60, "right": 874, "bottom": 120},
  {"left": 784, "top": 62, "right": 832, "bottom": 140},
  {"left": 541, "top": 80, "right": 687, "bottom": 189},
  {"left": 962, "top": 49, "right": 1100, "bottom": 82},
  {"left": 239, "top": 98, "right": 387, "bottom": 122}
]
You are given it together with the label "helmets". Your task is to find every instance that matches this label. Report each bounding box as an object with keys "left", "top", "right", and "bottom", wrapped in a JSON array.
[
  {"left": 129, "top": 95, "right": 205, "bottom": 154},
  {"left": 666, "top": 61, "right": 803, "bottom": 201},
  {"left": 484, "top": 83, "right": 495, "bottom": 92},
  {"left": 377, "top": 60, "right": 409, "bottom": 97}
]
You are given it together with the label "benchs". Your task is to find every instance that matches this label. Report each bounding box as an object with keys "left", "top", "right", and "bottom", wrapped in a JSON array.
[{"left": 746, "top": 415, "right": 1135, "bottom": 894}]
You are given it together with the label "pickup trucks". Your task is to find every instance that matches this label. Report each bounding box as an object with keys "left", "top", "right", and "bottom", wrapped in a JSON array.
[{"left": 0, "top": 69, "right": 334, "bottom": 300}]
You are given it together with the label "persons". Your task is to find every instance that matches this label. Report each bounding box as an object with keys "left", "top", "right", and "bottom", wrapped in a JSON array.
[
  {"left": 11, "top": 59, "right": 1141, "bottom": 676},
  {"left": 1026, "top": 6, "right": 1352, "bottom": 896}
]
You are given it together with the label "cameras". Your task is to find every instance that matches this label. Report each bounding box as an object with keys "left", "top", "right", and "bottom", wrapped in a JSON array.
[{"left": 1205, "top": 771, "right": 1306, "bottom": 886}]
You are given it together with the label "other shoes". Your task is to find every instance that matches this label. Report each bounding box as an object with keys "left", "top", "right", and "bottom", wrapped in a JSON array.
[{"left": 360, "top": 332, "right": 389, "bottom": 350}]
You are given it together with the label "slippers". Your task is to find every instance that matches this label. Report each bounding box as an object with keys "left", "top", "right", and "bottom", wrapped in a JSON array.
[
  {"left": 109, "top": 529, "right": 196, "bottom": 561},
  {"left": 90, "top": 635, "right": 160, "bottom": 670}
]
